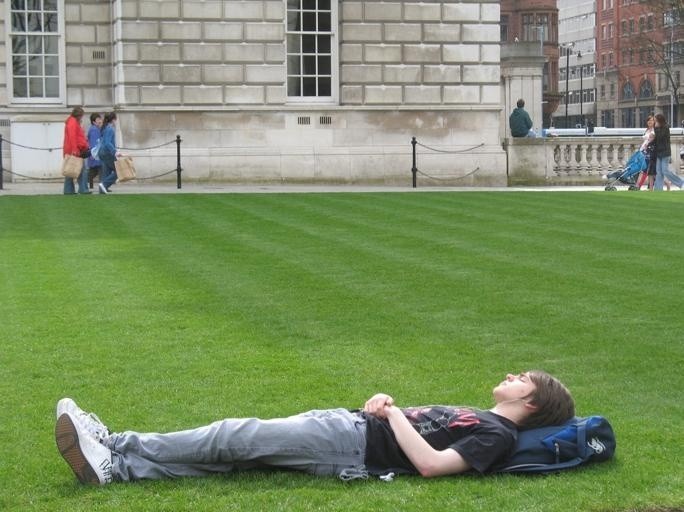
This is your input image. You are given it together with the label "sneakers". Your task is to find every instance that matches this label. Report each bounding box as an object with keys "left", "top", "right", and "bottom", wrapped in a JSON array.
[
  {"left": 55, "top": 396, "right": 110, "bottom": 445},
  {"left": 55, "top": 413, "right": 115, "bottom": 488},
  {"left": 99, "top": 182, "right": 108, "bottom": 194}
]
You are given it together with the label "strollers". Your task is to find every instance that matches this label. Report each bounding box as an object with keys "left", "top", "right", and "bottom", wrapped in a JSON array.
[{"left": 605, "top": 146, "right": 651, "bottom": 192}]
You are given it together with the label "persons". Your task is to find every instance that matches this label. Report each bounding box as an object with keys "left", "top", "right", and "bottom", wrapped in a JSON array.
[
  {"left": 606, "top": 166, "right": 630, "bottom": 183},
  {"left": 96, "top": 111, "right": 128, "bottom": 195},
  {"left": 633, "top": 112, "right": 684, "bottom": 192},
  {"left": 61, "top": 106, "right": 95, "bottom": 195},
  {"left": 53, "top": 369, "right": 576, "bottom": 492},
  {"left": 86, "top": 112, "right": 114, "bottom": 194},
  {"left": 507, "top": 98, "right": 539, "bottom": 138}
]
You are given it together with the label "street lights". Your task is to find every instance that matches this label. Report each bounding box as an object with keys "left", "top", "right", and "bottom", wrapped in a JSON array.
[{"left": 575, "top": 49, "right": 584, "bottom": 129}]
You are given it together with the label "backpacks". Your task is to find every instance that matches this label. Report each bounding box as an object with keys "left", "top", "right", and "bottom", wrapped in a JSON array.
[{"left": 494, "top": 415, "right": 616, "bottom": 473}]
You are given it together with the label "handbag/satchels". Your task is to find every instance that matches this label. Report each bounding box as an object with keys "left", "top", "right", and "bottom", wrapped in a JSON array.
[
  {"left": 115, "top": 153, "right": 137, "bottom": 182},
  {"left": 86, "top": 151, "right": 103, "bottom": 168},
  {"left": 62, "top": 151, "right": 82, "bottom": 179}
]
